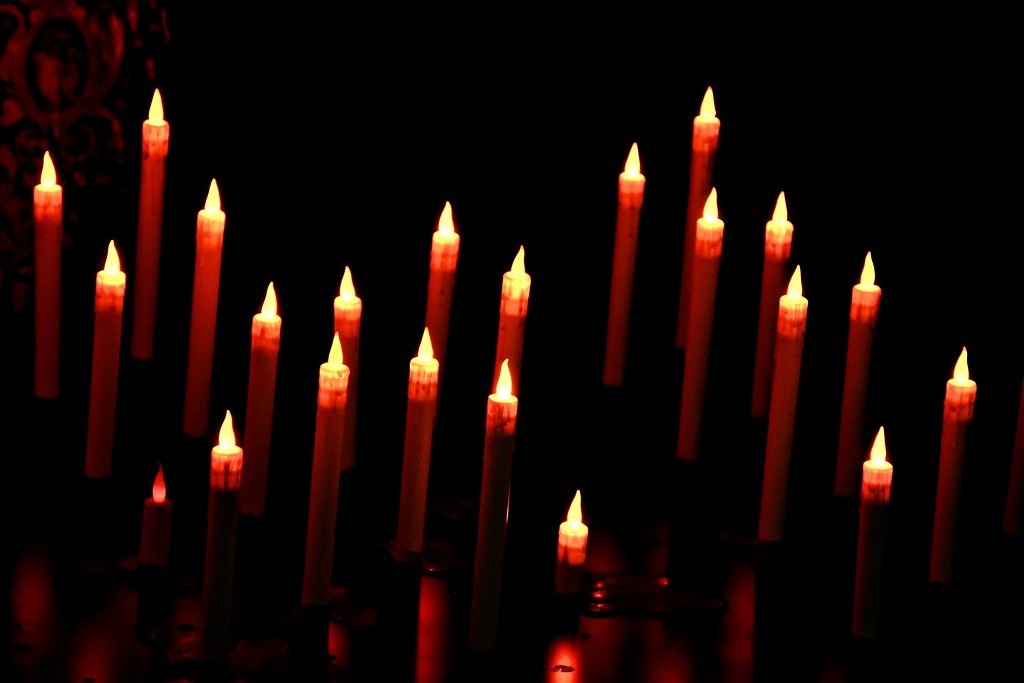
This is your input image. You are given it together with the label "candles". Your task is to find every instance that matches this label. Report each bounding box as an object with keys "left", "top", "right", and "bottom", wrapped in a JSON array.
[
  {"left": 757, "top": 265, "right": 811, "bottom": 541},
  {"left": 552, "top": 488, "right": 590, "bottom": 593},
  {"left": 198, "top": 410, "right": 246, "bottom": 657},
  {"left": 489, "top": 242, "right": 534, "bottom": 398},
  {"left": 84, "top": 239, "right": 127, "bottom": 476},
  {"left": 182, "top": 176, "right": 228, "bottom": 439},
  {"left": 332, "top": 265, "right": 365, "bottom": 471},
  {"left": 424, "top": 200, "right": 463, "bottom": 392},
  {"left": 33, "top": 149, "right": 64, "bottom": 398},
  {"left": 675, "top": 84, "right": 723, "bottom": 348},
  {"left": 675, "top": 186, "right": 725, "bottom": 460},
  {"left": 233, "top": 281, "right": 283, "bottom": 515},
  {"left": 835, "top": 250, "right": 1024, "bottom": 641},
  {"left": 751, "top": 190, "right": 797, "bottom": 416},
  {"left": 397, "top": 328, "right": 454, "bottom": 683},
  {"left": 602, "top": 140, "right": 649, "bottom": 386},
  {"left": 129, "top": 86, "right": 170, "bottom": 362},
  {"left": 135, "top": 463, "right": 173, "bottom": 644},
  {"left": 466, "top": 358, "right": 520, "bottom": 655},
  {"left": 302, "top": 331, "right": 352, "bottom": 602}
]
